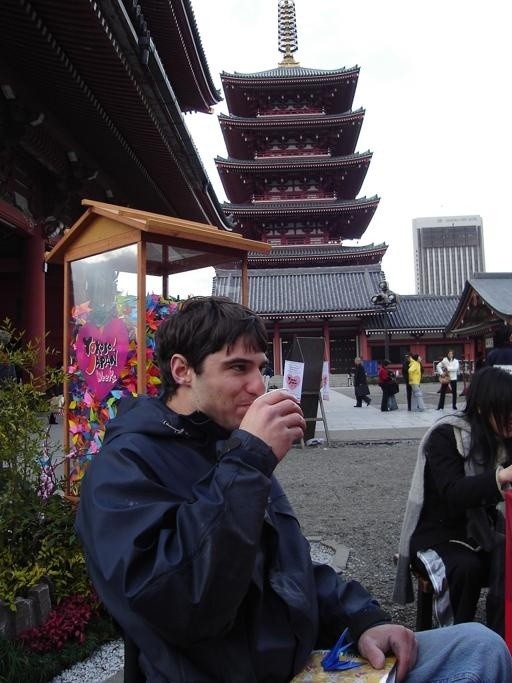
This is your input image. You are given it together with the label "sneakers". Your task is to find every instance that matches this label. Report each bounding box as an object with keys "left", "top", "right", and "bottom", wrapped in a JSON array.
[{"left": 354, "top": 400, "right": 458, "bottom": 411}]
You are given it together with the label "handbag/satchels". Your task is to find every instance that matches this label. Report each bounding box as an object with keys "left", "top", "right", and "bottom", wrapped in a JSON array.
[
  {"left": 438, "top": 373, "right": 450, "bottom": 384},
  {"left": 390, "top": 382, "right": 399, "bottom": 394},
  {"left": 357, "top": 382, "right": 370, "bottom": 397}
]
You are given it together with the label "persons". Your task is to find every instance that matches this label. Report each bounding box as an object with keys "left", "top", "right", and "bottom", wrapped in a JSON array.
[
  {"left": 378, "top": 358, "right": 391, "bottom": 411},
  {"left": 384, "top": 370, "right": 399, "bottom": 410},
  {"left": 353, "top": 356, "right": 371, "bottom": 408},
  {"left": 435, "top": 347, "right": 458, "bottom": 410},
  {"left": 485, "top": 325, "right": 512, "bottom": 367},
  {"left": 402, "top": 352, "right": 424, "bottom": 411},
  {"left": 473, "top": 351, "right": 485, "bottom": 371},
  {"left": 75, "top": 292, "right": 512, "bottom": 682},
  {"left": 406, "top": 352, "right": 426, "bottom": 410},
  {"left": 385, "top": 364, "right": 511, "bottom": 632}
]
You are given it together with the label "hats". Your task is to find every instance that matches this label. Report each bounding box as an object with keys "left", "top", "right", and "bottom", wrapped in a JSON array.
[
  {"left": 412, "top": 354, "right": 418, "bottom": 361},
  {"left": 482, "top": 348, "right": 512, "bottom": 377}
]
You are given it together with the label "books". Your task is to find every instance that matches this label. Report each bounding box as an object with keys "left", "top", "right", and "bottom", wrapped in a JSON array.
[{"left": 289, "top": 647, "right": 398, "bottom": 682}]
